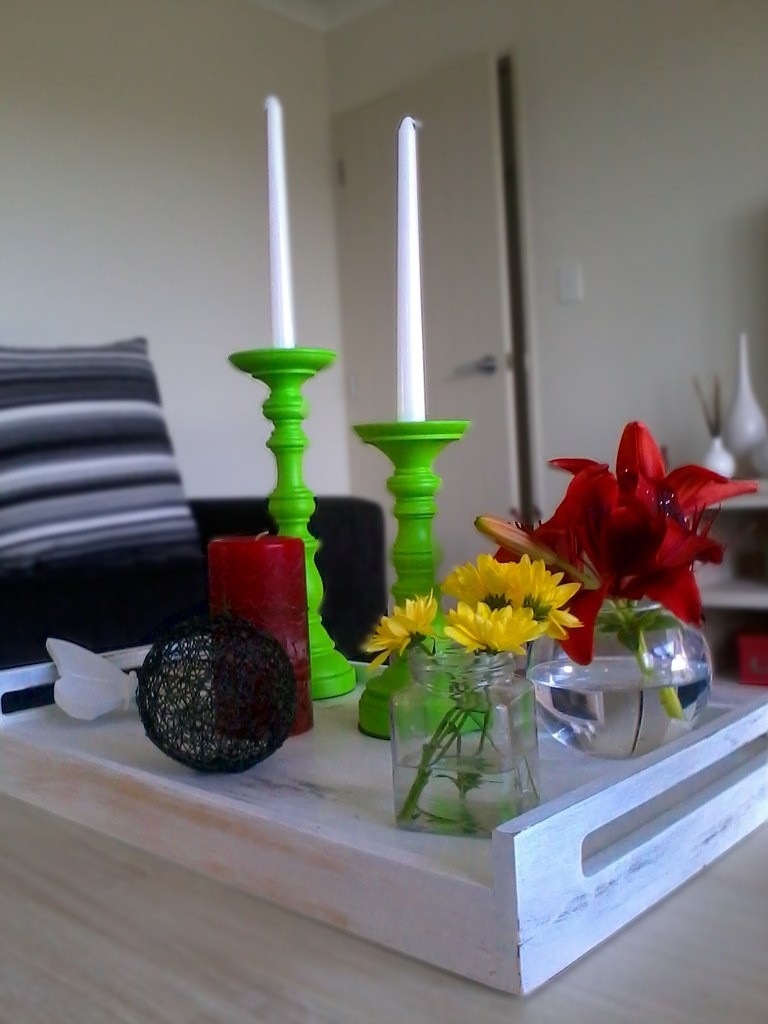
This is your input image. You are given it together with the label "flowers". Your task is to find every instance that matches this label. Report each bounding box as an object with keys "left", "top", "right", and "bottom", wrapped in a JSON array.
[
  {"left": 365, "top": 546, "right": 587, "bottom": 787},
  {"left": 486, "top": 419, "right": 756, "bottom": 716}
]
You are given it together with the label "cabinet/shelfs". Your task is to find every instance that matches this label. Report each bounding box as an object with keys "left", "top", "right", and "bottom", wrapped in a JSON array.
[{"left": 693, "top": 480, "right": 768, "bottom": 709}]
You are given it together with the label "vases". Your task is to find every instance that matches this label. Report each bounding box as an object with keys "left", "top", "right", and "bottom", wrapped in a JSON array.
[
  {"left": 520, "top": 593, "right": 719, "bottom": 759},
  {"left": 388, "top": 647, "right": 543, "bottom": 836},
  {"left": 700, "top": 434, "right": 737, "bottom": 480}
]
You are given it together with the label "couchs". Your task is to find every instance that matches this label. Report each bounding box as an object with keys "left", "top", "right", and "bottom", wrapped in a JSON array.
[{"left": 187, "top": 495, "right": 386, "bottom": 661}]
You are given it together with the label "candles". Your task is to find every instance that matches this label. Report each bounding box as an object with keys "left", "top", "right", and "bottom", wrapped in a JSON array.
[
  {"left": 397, "top": 112, "right": 426, "bottom": 419},
  {"left": 262, "top": 92, "right": 296, "bottom": 351},
  {"left": 211, "top": 530, "right": 313, "bottom": 734}
]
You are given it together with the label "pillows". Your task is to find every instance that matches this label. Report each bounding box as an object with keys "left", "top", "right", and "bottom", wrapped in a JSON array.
[{"left": 1, "top": 333, "right": 210, "bottom": 716}]
